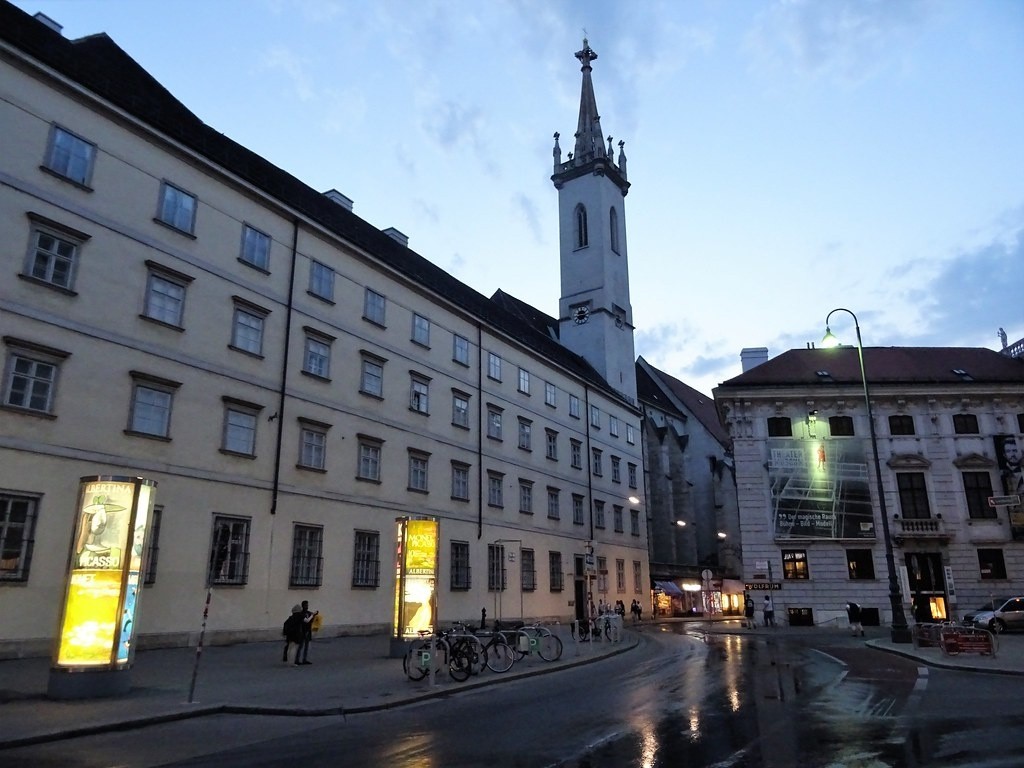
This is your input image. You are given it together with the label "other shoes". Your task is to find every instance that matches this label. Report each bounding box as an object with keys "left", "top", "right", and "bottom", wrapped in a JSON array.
[
  {"left": 295, "top": 662, "right": 302, "bottom": 665},
  {"left": 303, "top": 661, "right": 312, "bottom": 664}
]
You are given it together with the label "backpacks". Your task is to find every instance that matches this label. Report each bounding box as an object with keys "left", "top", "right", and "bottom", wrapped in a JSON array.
[{"left": 850, "top": 603, "right": 858, "bottom": 614}]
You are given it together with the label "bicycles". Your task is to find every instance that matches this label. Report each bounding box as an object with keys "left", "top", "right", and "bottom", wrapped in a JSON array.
[
  {"left": 451, "top": 619, "right": 516, "bottom": 674},
  {"left": 504, "top": 618, "right": 564, "bottom": 663},
  {"left": 404, "top": 616, "right": 489, "bottom": 682},
  {"left": 572, "top": 610, "right": 622, "bottom": 643}
]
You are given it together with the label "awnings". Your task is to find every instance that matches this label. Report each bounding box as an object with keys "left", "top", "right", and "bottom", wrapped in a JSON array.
[
  {"left": 654, "top": 582, "right": 683, "bottom": 596},
  {"left": 721, "top": 580, "right": 746, "bottom": 594},
  {"left": 679, "top": 577, "right": 702, "bottom": 592}
]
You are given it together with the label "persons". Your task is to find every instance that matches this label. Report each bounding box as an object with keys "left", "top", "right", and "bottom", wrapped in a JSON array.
[
  {"left": 591, "top": 599, "right": 642, "bottom": 623},
  {"left": 744, "top": 594, "right": 757, "bottom": 630},
  {"left": 846, "top": 601, "right": 865, "bottom": 637},
  {"left": 816, "top": 444, "right": 825, "bottom": 471},
  {"left": 282, "top": 600, "right": 319, "bottom": 667},
  {"left": 763, "top": 596, "right": 774, "bottom": 628},
  {"left": 1000, "top": 438, "right": 1024, "bottom": 513}
]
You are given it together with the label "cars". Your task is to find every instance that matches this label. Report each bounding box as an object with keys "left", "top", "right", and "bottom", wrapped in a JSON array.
[{"left": 965, "top": 594, "right": 1024, "bottom": 635}]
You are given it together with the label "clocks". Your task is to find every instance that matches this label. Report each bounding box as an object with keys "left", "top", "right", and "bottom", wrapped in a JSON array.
[{"left": 573, "top": 305, "right": 590, "bottom": 325}]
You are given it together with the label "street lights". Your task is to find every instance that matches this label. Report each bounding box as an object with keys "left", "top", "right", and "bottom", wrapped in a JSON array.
[{"left": 821, "top": 308, "right": 914, "bottom": 644}]
